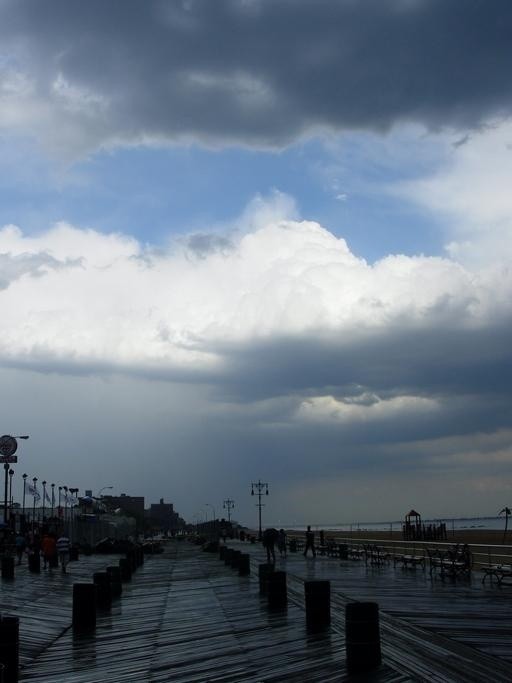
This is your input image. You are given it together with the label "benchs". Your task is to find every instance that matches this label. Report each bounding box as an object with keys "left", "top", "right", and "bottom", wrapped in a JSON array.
[{"left": 313, "top": 539, "right": 512, "bottom": 592}]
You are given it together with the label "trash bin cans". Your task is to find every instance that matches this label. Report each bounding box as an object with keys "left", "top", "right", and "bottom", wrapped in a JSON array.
[
  {"left": 339, "top": 543, "right": 348, "bottom": 560},
  {"left": 251, "top": 537, "right": 255, "bottom": 543},
  {"left": 29, "top": 555, "right": 40, "bottom": 571},
  {"left": 1, "top": 557, "right": 15, "bottom": 580},
  {"left": 290, "top": 540, "right": 298, "bottom": 553}
]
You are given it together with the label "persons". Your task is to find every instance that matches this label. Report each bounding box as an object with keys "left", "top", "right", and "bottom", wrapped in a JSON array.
[
  {"left": 221, "top": 526, "right": 246, "bottom": 543},
  {"left": 263, "top": 527, "right": 288, "bottom": 562},
  {"left": 103, "top": 534, "right": 140, "bottom": 545},
  {"left": 302, "top": 525, "right": 316, "bottom": 559},
  {"left": 15, "top": 532, "right": 72, "bottom": 574}
]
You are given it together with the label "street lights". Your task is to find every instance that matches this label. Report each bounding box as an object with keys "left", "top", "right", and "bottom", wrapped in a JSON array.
[
  {"left": 206, "top": 503, "right": 215, "bottom": 528},
  {"left": 96, "top": 486, "right": 113, "bottom": 515},
  {"left": 251, "top": 479, "right": 268, "bottom": 542},
  {"left": 223, "top": 496, "right": 234, "bottom": 521}
]
